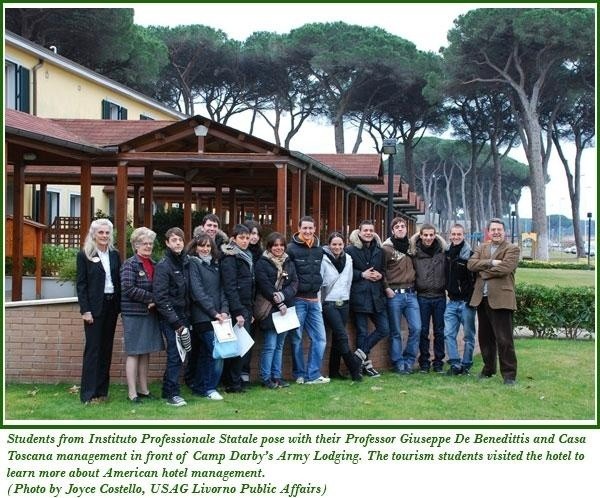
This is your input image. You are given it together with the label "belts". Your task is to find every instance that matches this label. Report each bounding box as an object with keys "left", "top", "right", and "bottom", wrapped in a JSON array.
[
  {"left": 329, "top": 300, "right": 350, "bottom": 306},
  {"left": 394, "top": 288, "right": 416, "bottom": 294}
]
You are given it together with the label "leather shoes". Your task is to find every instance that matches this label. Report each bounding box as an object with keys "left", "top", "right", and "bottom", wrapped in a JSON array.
[
  {"left": 127, "top": 395, "right": 142, "bottom": 404},
  {"left": 137, "top": 390, "right": 158, "bottom": 400}
]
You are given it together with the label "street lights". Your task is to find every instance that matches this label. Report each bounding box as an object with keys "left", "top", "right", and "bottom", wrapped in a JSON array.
[
  {"left": 507, "top": 208, "right": 521, "bottom": 256},
  {"left": 583, "top": 211, "right": 597, "bottom": 267},
  {"left": 379, "top": 136, "right": 401, "bottom": 240}
]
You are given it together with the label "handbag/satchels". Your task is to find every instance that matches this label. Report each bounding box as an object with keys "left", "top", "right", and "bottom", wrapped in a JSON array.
[
  {"left": 181, "top": 328, "right": 192, "bottom": 352},
  {"left": 213, "top": 337, "right": 238, "bottom": 359},
  {"left": 253, "top": 293, "right": 272, "bottom": 320}
]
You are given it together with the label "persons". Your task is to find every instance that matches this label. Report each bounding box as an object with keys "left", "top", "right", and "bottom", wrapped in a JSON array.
[{"left": 74, "top": 216, "right": 522, "bottom": 404}]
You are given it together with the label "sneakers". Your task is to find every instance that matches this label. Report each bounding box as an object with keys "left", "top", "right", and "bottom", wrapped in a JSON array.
[
  {"left": 207, "top": 391, "right": 223, "bottom": 400},
  {"left": 89, "top": 395, "right": 109, "bottom": 404},
  {"left": 167, "top": 395, "right": 187, "bottom": 407},
  {"left": 448, "top": 366, "right": 471, "bottom": 378},
  {"left": 399, "top": 367, "right": 414, "bottom": 375},
  {"left": 503, "top": 378, "right": 514, "bottom": 385},
  {"left": 297, "top": 376, "right": 304, "bottom": 384},
  {"left": 477, "top": 374, "right": 494, "bottom": 378},
  {"left": 433, "top": 366, "right": 443, "bottom": 373},
  {"left": 306, "top": 376, "right": 331, "bottom": 385},
  {"left": 419, "top": 366, "right": 430, "bottom": 373},
  {"left": 265, "top": 376, "right": 290, "bottom": 390}
]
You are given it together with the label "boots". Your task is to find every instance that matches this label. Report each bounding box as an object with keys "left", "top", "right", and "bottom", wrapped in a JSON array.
[
  {"left": 352, "top": 349, "right": 381, "bottom": 379},
  {"left": 329, "top": 370, "right": 346, "bottom": 379}
]
